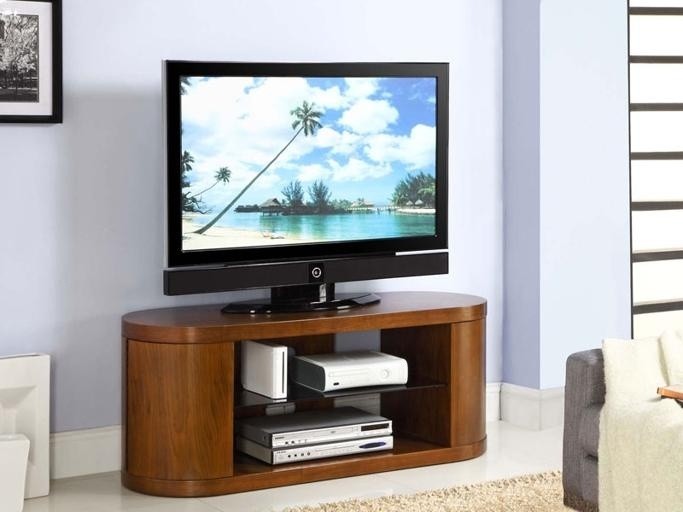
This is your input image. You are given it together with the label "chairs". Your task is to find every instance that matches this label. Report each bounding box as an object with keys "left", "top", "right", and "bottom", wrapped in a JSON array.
[{"left": 563, "top": 351, "right": 604, "bottom": 512}]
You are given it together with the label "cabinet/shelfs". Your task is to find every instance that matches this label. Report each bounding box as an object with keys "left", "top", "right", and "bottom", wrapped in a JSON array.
[{"left": 121, "top": 291, "right": 487, "bottom": 497}]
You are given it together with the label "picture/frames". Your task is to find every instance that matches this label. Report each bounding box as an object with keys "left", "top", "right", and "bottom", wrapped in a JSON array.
[{"left": 0, "top": 0, "right": 62, "bottom": 124}]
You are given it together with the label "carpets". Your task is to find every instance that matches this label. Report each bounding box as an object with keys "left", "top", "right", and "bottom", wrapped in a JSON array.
[{"left": 280, "top": 468, "right": 598, "bottom": 512}]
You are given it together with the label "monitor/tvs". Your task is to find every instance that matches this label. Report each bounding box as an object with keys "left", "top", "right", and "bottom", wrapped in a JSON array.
[{"left": 160, "top": 60, "right": 449, "bottom": 315}]
